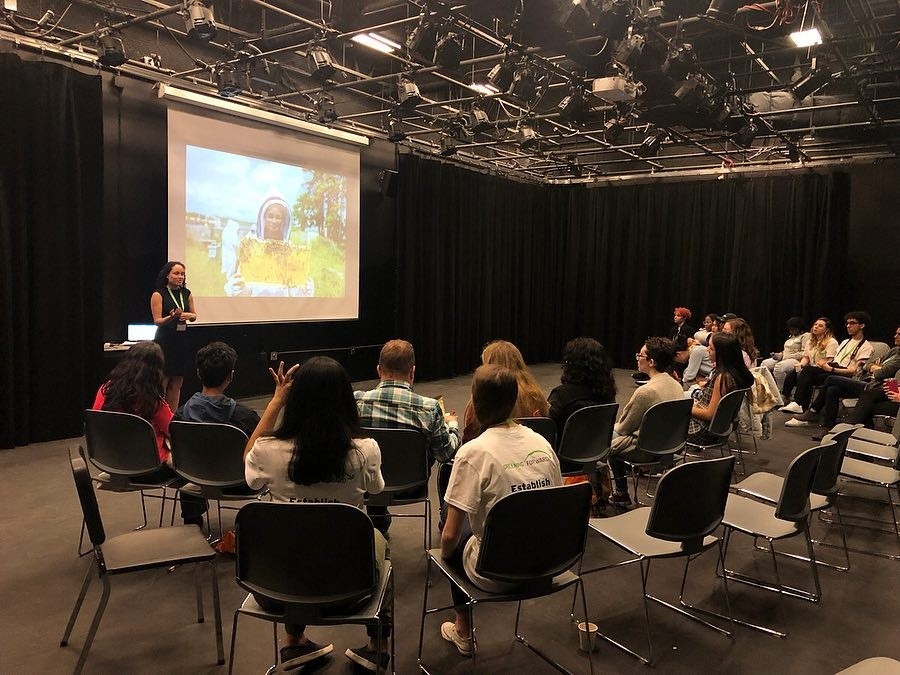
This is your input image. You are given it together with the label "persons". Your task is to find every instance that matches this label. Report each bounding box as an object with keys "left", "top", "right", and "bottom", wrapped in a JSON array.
[
  {"left": 760, "top": 311, "right": 900, "bottom": 446},
  {"left": 151, "top": 261, "right": 196, "bottom": 413},
  {"left": 222, "top": 197, "right": 315, "bottom": 297},
  {"left": 244, "top": 356, "right": 392, "bottom": 673},
  {"left": 91, "top": 305, "right": 757, "bottom": 541},
  {"left": 441, "top": 365, "right": 565, "bottom": 655}
]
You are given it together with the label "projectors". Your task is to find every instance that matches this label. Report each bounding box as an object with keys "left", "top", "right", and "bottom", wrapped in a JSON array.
[{"left": 592, "top": 76, "right": 636, "bottom": 102}]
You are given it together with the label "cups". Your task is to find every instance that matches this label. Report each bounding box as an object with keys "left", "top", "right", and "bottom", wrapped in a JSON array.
[{"left": 577, "top": 622, "right": 600, "bottom": 652}]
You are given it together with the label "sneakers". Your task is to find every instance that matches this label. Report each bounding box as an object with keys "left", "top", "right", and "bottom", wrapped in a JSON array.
[
  {"left": 280, "top": 637, "right": 334, "bottom": 670},
  {"left": 345, "top": 644, "right": 391, "bottom": 673},
  {"left": 784, "top": 417, "right": 809, "bottom": 427},
  {"left": 777, "top": 401, "right": 804, "bottom": 414},
  {"left": 441, "top": 621, "right": 478, "bottom": 656}
]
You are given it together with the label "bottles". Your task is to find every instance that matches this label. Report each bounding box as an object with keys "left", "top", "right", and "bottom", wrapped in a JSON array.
[{"left": 888, "top": 378, "right": 898, "bottom": 393}]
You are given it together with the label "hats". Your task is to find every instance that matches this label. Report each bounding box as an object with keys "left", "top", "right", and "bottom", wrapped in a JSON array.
[{"left": 714, "top": 312, "right": 737, "bottom": 322}]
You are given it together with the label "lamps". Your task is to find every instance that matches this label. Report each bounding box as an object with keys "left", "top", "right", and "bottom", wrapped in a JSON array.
[{"left": 98, "top": 0, "right": 825, "bottom": 151}]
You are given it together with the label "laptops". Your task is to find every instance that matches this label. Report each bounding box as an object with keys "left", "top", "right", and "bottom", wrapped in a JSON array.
[{"left": 121, "top": 320, "right": 159, "bottom": 346}]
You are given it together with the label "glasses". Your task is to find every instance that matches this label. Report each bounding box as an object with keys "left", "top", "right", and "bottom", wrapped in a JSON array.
[
  {"left": 635, "top": 353, "right": 650, "bottom": 361},
  {"left": 703, "top": 321, "right": 713, "bottom": 325},
  {"left": 844, "top": 321, "right": 859, "bottom": 327}
]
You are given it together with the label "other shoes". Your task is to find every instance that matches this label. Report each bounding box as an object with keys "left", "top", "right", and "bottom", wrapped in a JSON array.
[
  {"left": 608, "top": 493, "right": 632, "bottom": 506},
  {"left": 779, "top": 394, "right": 790, "bottom": 406},
  {"left": 793, "top": 407, "right": 820, "bottom": 422},
  {"left": 812, "top": 426, "right": 831, "bottom": 440}
]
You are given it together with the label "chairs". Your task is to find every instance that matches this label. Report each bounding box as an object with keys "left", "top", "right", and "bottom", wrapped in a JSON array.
[{"left": 67, "top": 357, "right": 900, "bottom": 675}]
